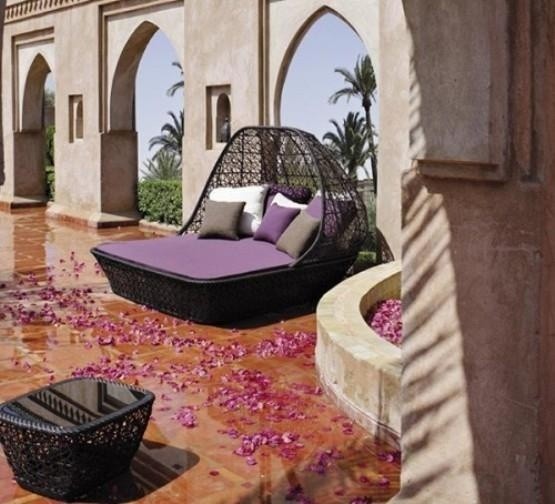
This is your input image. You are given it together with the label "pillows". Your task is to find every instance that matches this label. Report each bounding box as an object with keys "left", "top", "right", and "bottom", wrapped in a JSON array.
[{"left": 196, "top": 183, "right": 357, "bottom": 258}]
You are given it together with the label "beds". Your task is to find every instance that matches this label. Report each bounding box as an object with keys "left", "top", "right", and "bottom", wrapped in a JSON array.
[{"left": 88, "top": 125, "right": 367, "bottom": 326}]
